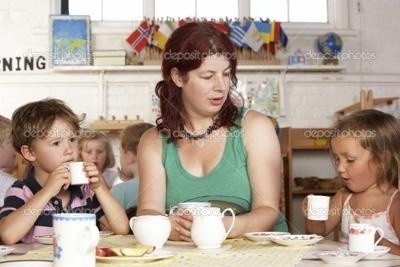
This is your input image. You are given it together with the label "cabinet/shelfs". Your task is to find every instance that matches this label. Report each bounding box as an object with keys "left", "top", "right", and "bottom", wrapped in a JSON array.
[{"left": 290, "top": 127, "right": 346, "bottom": 235}]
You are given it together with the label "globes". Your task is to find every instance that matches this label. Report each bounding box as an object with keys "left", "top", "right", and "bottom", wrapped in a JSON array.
[{"left": 316, "top": 33, "right": 343, "bottom": 65}]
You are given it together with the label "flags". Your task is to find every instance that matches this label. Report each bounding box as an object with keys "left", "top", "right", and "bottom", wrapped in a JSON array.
[{"left": 126, "top": 16, "right": 288, "bottom": 60}]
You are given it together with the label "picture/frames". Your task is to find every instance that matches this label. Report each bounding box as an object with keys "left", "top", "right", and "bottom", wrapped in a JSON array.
[{"left": 48, "top": 14, "right": 90, "bottom": 66}]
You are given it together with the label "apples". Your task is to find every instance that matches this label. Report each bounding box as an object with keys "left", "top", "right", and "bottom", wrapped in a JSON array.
[{"left": 96, "top": 242, "right": 157, "bottom": 257}]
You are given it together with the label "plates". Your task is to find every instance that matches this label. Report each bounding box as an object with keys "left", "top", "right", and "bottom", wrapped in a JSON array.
[
  {"left": 271, "top": 234, "right": 324, "bottom": 246},
  {"left": 343, "top": 246, "right": 391, "bottom": 257},
  {"left": 96, "top": 250, "right": 173, "bottom": 263},
  {"left": 0, "top": 247, "right": 15, "bottom": 256},
  {"left": 33, "top": 235, "right": 53, "bottom": 245},
  {"left": 244, "top": 231, "right": 291, "bottom": 242},
  {"left": 317, "top": 252, "right": 366, "bottom": 264}
]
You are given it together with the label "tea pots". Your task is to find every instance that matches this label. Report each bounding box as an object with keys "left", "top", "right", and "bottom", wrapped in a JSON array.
[{"left": 190, "top": 207, "right": 235, "bottom": 250}]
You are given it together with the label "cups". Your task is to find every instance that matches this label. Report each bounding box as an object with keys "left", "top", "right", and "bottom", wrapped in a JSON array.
[
  {"left": 170, "top": 201, "right": 210, "bottom": 215},
  {"left": 306, "top": 195, "right": 330, "bottom": 221},
  {"left": 64, "top": 162, "right": 89, "bottom": 185},
  {"left": 129, "top": 215, "right": 172, "bottom": 249},
  {"left": 53, "top": 212, "right": 99, "bottom": 267},
  {"left": 349, "top": 224, "right": 384, "bottom": 252}
]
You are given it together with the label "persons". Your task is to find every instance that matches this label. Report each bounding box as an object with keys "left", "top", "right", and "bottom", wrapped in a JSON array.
[
  {"left": 302, "top": 109, "right": 400, "bottom": 258},
  {"left": 0, "top": 97, "right": 131, "bottom": 245},
  {"left": 0, "top": 115, "right": 19, "bottom": 208},
  {"left": 131, "top": 22, "right": 282, "bottom": 242},
  {"left": 78, "top": 132, "right": 118, "bottom": 189},
  {"left": 109, "top": 123, "right": 157, "bottom": 234}
]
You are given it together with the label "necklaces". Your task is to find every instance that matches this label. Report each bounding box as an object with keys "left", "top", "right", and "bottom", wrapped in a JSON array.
[{"left": 180, "top": 123, "right": 221, "bottom": 140}]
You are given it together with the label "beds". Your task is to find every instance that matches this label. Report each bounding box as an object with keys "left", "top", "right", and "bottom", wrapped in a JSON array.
[{"left": 0, "top": 234, "right": 400, "bottom": 267}]
